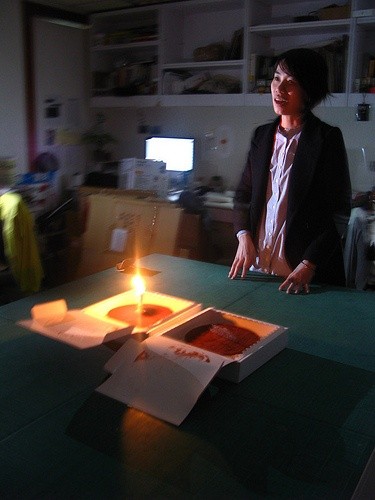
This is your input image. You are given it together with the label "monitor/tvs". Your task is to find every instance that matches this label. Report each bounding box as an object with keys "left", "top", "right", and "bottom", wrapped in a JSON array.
[{"left": 144, "top": 134, "right": 195, "bottom": 171}]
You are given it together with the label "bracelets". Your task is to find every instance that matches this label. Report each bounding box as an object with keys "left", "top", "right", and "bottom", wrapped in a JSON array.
[{"left": 301, "top": 261, "right": 316, "bottom": 273}]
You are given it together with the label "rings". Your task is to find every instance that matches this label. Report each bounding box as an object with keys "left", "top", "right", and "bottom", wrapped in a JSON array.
[{"left": 291, "top": 281, "right": 295, "bottom": 286}]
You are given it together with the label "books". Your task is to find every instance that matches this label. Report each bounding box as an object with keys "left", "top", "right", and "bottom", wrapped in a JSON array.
[
  {"left": 316, "top": 47, "right": 345, "bottom": 93},
  {"left": 92, "top": 63, "right": 158, "bottom": 95}
]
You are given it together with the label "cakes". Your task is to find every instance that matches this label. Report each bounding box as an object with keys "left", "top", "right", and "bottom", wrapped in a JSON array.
[
  {"left": 107, "top": 304, "right": 172, "bottom": 328},
  {"left": 184, "top": 325, "right": 260, "bottom": 360}
]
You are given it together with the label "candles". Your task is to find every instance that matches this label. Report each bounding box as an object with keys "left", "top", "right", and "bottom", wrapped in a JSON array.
[{"left": 128, "top": 273, "right": 147, "bottom": 314}]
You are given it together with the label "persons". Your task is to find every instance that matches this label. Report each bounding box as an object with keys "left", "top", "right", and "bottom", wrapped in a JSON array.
[{"left": 228, "top": 48, "right": 352, "bottom": 294}]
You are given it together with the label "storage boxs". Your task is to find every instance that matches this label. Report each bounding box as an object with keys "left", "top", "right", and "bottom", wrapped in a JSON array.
[
  {"left": 96, "top": 306, "right": 288, "bottom": 426},
  {"left": 14, "top": 287, "right": 204, "bottom": 356}
]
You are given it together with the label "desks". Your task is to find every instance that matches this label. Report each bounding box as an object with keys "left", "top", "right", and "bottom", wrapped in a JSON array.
[
  {"left": 0, "top": 252, "right": 375, "bottom": 500},
  {"left": 73, "top": 193, "right": 200, "bottom": 283},
  {"left": 77, "top": 184, "right": 240, "bottom": 269}
]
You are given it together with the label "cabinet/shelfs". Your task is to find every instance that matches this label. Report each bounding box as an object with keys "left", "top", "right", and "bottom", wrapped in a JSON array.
[
  {"left": 85, "top": 0, "right": 375, "bottom": 108},
  {"left": 0, "top": 188, "right": 77, "bottom": 307}
]
